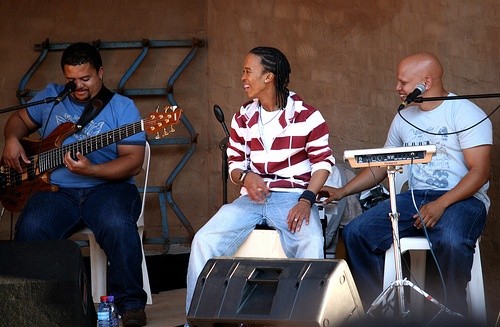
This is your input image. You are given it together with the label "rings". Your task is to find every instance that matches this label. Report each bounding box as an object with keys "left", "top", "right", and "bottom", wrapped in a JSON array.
[
  {"left": 66, "top": 164, "right": 68, "bottom": 167},
  {"left": 294, "top": 218, "right": 298, "bottom": 221}
]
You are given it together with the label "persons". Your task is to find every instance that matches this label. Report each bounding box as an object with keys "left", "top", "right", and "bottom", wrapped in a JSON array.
[
  {"left": 1, "top": 41, "right": 148, "bottom": 327},
  {"left": 186, "top": 45, "right": 335, "bottom": 326},
  {"left": 316, "top": 52, "right": 493, "bottom": 327}
]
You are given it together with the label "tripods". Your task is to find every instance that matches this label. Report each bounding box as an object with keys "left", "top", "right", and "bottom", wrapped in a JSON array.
[{"left": 344, "top": 145, "right": 473, "bottom": 327}]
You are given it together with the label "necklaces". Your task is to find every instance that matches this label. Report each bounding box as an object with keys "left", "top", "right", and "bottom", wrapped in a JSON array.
[{"left": 259, "top": 101, "right": 281, "bottom": 135}]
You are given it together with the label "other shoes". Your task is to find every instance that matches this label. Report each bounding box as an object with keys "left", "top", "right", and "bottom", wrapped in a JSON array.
[{"left": 122, "top": 309, "right": 146, "bottom": 327}]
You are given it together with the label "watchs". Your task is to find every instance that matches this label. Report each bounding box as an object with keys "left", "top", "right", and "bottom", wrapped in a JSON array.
[{"left": 239, "top": 169, "right": 251, "bottom": 183}]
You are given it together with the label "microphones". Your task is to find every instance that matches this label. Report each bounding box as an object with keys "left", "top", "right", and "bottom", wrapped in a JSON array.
[
  {"left": 55, "top": 80, "right": 78, "bottom": 105},
  {"left": 398, "top": 84, "right": 426, "bottom": 111}
]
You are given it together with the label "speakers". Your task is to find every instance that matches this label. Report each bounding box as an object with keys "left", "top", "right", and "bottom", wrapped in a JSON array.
[
  {"left": 0, "top": 237, "right": 98, "bottom": 327},
  {"left": 185, "top": 256, "right": 365, "bottom": 327}
]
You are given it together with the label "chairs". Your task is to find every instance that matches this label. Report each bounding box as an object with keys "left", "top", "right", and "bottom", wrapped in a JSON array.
[
  {"left": 77, "top": 139, "right": 152, "bottom": 306},
  {"left": 382, "top": 236, "right": 489, "bottom": 327}
]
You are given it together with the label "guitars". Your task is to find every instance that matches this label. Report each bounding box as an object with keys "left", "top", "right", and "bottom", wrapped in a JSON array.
[{"left": 0, "top": 105, "right": 183, "bottom": 212}]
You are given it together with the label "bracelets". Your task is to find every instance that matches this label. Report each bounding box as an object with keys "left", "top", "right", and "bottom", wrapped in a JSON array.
[{"left": 297, "top": 189, "right": 316, "bottom": 208}]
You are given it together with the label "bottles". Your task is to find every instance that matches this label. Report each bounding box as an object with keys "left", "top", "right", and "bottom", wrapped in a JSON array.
[
  {"left": 96, "top": 296, "right": 112, "bottom": 327},
  {"left": 107, "top": 296, "right": 119, "bottom": 327}
]
